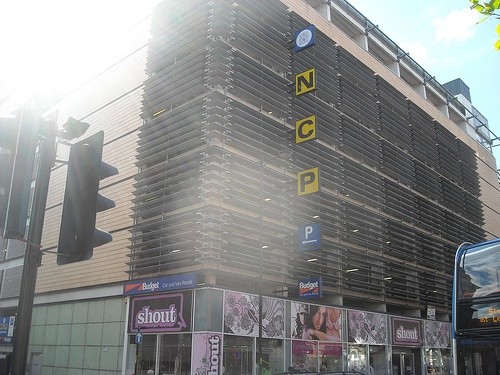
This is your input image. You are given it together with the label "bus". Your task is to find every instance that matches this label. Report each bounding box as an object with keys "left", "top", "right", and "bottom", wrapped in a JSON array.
[{"left": 452, "top": 235, "right": 500, "bottom": 375}]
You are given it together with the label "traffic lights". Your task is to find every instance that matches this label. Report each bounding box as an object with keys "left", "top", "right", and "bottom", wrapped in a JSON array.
[{"left": 57, "top": 130, "right": 119, "bottom": 268}]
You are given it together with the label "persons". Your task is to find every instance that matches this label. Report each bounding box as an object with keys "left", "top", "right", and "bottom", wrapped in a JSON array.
[{"left": 291, "top": 302, "right": 342, "bottom": 342}]
